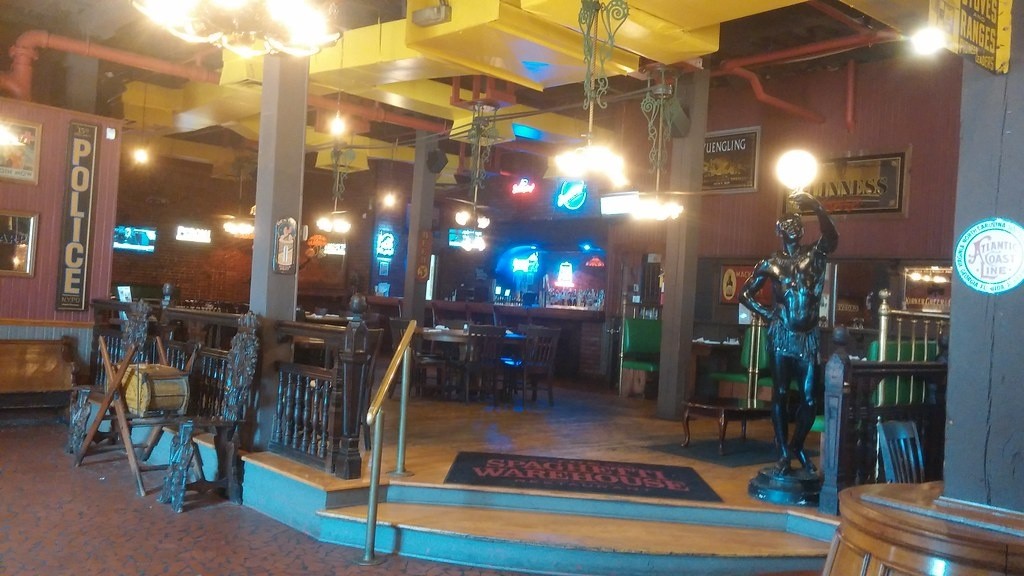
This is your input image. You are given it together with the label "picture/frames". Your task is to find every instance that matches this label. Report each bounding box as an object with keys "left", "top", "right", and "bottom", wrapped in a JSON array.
[
  {"left": 702, "top": 124, "right": 762, "bottom": 194},
  {"left": 775, "top": 143, "right": 914, "bottom": 222},
  {"left": 0, "top": 118, "right": 42, "bottom": 186},
  {"left": 0, "top": 209, "right": 40, "bottom": 278}
]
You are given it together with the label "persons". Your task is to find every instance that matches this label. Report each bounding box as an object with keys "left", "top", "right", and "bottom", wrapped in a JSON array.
[{"left": 737, "top": 190, "right": 839, "bottom": 476}]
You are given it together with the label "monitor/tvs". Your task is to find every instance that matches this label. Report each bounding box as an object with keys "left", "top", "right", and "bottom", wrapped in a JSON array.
[
  {"left": 113, "top": 226, "right": 158, "bottom": 255},
  {"left": 447, "top": 227, "right": 483, "bottom": 248}
]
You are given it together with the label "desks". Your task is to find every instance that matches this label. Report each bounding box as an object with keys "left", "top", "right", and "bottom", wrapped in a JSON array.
[
  {"left": 681, "top": 397, "right": 778, "bottom": 456},
  {"left": 690, "top": 337, "right": 742, "bottom": 398},
  {"left": 422, "top": 327, "right": 530, "bottom": 396}
]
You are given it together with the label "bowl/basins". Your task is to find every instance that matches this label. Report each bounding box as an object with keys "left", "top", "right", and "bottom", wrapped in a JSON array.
[{"left": 315, "top": 307, "right": 328, "bottom": 315}]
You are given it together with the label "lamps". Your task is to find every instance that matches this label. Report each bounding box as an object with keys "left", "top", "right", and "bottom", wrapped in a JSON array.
[
  {"left": 633, "top": 68, "right": 683, "bottom": 225},
  {"left": 317, "top": 151, "right": 354, "bottom": 233},
  {"left": 222, "top": 160, "right": 254, "bottom": 238},
  {"left": 330, "top": 40, "right": 346, "bottom": 135},
  {"left": 453, "top": 100, "right": 503, "bottom": 251},
  {"left": 557, "top": 0, "right": 629, "bottom": 189}
]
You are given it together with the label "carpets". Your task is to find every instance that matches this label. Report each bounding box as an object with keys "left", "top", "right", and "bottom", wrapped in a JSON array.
[{"left": 443, "top": 451, "right": 724, "bottom": 504}]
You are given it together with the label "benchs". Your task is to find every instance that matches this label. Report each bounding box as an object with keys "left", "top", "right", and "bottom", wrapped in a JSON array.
[
  {"left": 710, "top": 327, "right": 753, "bottom": 397},
  {"left": 757, "top": 328, "right": 800, "bottom": 404},
  {"left": 111, "top": 282, "right": 179, "bottom": 307},
  {"left": 621, "top": 318, "right": 662, "bottom": 401},
  {"left": 808, "top": 340, "right": 938, "bottom": 435}
]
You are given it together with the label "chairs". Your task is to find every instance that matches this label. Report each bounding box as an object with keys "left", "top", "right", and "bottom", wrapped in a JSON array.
[
  {"left": 464, "top": 300, "right": 471, "bottom": 321},
  {"left": 431, "top": 302, "right": 442, "bottom": 356},
  {"left": 450, "top": 326, "right": 505, "bottom": 407},
  {"left": 503, "top": 327, "right": 562, "bottom": 407},
  {"left": 387, "top": 316, "right": 445, "bottom": 401},
  {"left": 397, "top": 300, "right": 405, "bottom": 340},
  {"left": 491, "top": 306, "right": 501, "bottom": 325},
  {"left": 525, "top": 308, "right": 533, "bottom": 324},
  {"left": 873, "top": 420, "right": 929, "bottom": 484},
  {"left": 312, "top": 327, "right": 384, "bottom": 451}
]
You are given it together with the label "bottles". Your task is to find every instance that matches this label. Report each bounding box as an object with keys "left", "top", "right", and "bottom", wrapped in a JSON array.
[{"left": 548, "top": 288, "right": 598, "bottom": 307}]
